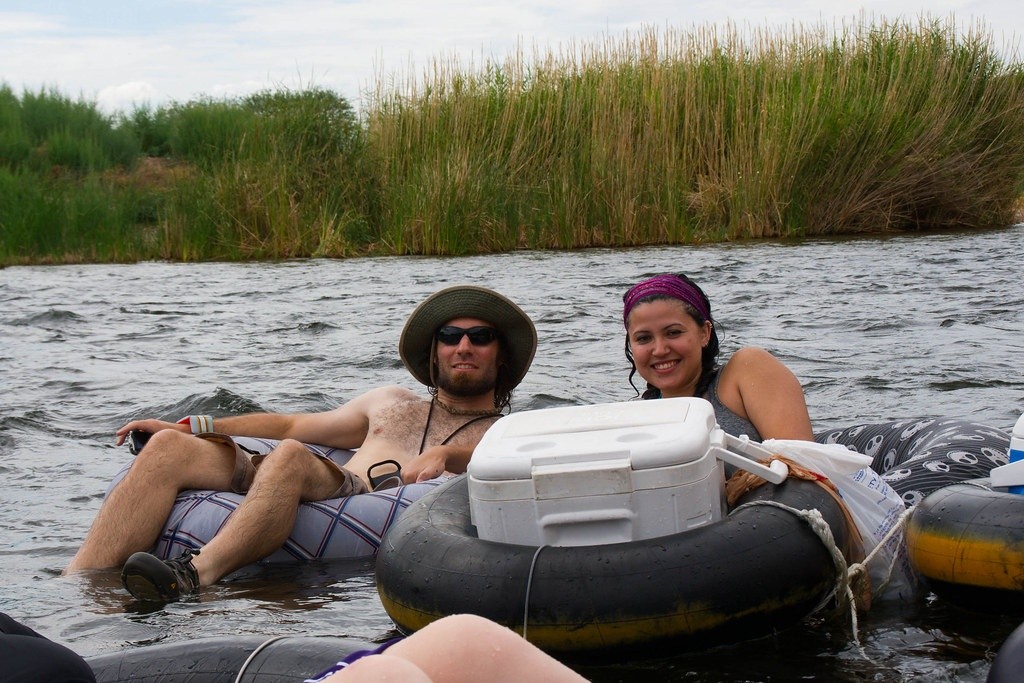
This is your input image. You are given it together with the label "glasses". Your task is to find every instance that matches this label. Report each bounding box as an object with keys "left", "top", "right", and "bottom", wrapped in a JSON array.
[{"left": 437, "top": 324, "right": 493, "bottom": 348}]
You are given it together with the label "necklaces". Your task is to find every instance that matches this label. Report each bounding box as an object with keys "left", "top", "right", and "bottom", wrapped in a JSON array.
[{"left": 419, "top": 398, "right": 504, "bottom": 454}]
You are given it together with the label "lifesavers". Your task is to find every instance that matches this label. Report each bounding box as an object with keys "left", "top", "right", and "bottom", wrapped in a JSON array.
[
  {"left": 902, "top": 470, "right": 1024, "bottom": 619},
  {"left": 99, "top": 433, "right": 452, "bottom": 584},
  {"left": 986, "top": 619, "right": 1023, "bottom": 683},
  {"left": 367, "top": 441, "right": 859, "bottom": 677},
  {"left": 83, "top": 632, "right": 387, "bottom": 683}
]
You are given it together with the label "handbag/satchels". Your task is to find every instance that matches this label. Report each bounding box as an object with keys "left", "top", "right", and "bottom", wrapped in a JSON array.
[{"left": 763, "top": 440, "right": 905, "bottom": 585}]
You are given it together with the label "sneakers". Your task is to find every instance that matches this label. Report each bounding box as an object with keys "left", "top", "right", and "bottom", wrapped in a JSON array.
[{"left": 122, "top": 550, "right": 200, "bottom": 604}]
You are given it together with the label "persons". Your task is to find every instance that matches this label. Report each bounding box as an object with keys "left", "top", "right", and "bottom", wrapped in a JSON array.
[
  {"left": 304, "top": 614, "right": 593, "bottom": 683},
  {"left": 64, "top": 285, "right": 539, "bottom": 601},
  {"left": 621, "top": 274, "right": 815, "bottom": 478}
]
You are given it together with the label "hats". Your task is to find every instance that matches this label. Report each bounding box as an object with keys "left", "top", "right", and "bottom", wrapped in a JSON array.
[{"left": 398, "top": 285, "right": 537, "bottom": 394}]
